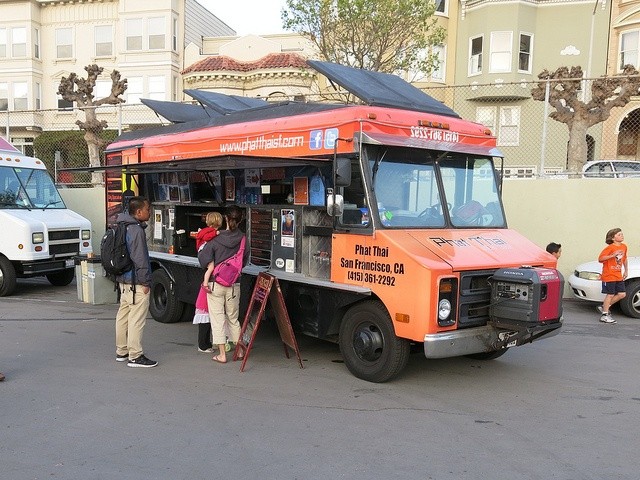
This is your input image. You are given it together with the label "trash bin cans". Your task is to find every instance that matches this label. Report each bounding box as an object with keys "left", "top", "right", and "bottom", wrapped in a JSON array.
[
  {"left": 87, "top": 258, "right": 118, "bottom": 305},
  {"left": 72, "top": 257, "right": 83, "bottom": 301},
  {"left": 80, "top": 258, "right": 88, "bottom": 303}
]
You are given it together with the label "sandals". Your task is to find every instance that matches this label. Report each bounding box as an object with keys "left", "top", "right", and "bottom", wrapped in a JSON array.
[{"left": 201, "top": 283, "right": 211, "bottom": 294}]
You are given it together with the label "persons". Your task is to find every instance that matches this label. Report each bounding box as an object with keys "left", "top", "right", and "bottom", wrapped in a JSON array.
[
  {"left": 198, "top": 206, "right": 248, "bottom": 363},
  {"left": 193, "top": 283, "right": 214, "bottom": 352},
  {"left": 597, "top": 228, "right": 628, "bottom": 323},
  {"left": 196, "top": 213, "right": 222, "bottom": 290},
  {"left": 100, "top": 196, "right": 158, "bottom": 368},
  {"left": 546, "top": 242, "right": 561, "bottom": 259}
]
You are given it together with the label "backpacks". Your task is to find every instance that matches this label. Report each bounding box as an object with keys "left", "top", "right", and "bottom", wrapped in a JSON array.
[{"left": 100, "top": 221, "right": 143, "bottom": 305}]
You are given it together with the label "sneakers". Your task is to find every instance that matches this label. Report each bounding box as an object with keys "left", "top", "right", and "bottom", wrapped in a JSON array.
[
  {"left": 197, "top": 347, "right": 215, "bottom": 353},
  {"left": 116, "top": 353, "right": 129, "bottom": 362},
  {"left": 596, "top": 305, "right": 618, "bottom": 324},
  {"left": 127, "top": 354, "right": 159, "bottom": 368}
]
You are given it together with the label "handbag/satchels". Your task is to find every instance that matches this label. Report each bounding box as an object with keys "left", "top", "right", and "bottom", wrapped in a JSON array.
[{"left": 212, "top": 235, "right": 247, "bottom": 297}]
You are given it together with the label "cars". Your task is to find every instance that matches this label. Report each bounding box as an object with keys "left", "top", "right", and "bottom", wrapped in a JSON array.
[
  {"left": 554, "top": 159, "right": 640, "bottom": 177},
  {"left": 569, "top": 257, "right": 640, "bottom": 319}
]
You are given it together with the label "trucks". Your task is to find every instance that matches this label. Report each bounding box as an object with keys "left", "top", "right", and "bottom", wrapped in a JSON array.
[
  {"left": 1, "top": 155, "right": 91, "bottom": 296},
  {"left": 105, "top": 61, "right": 565, "bottom": 383}
]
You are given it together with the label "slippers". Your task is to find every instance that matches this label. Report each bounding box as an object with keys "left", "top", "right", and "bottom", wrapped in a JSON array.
[{"left": 211, "top": 354, "right": 227, "bottom": 363}]
